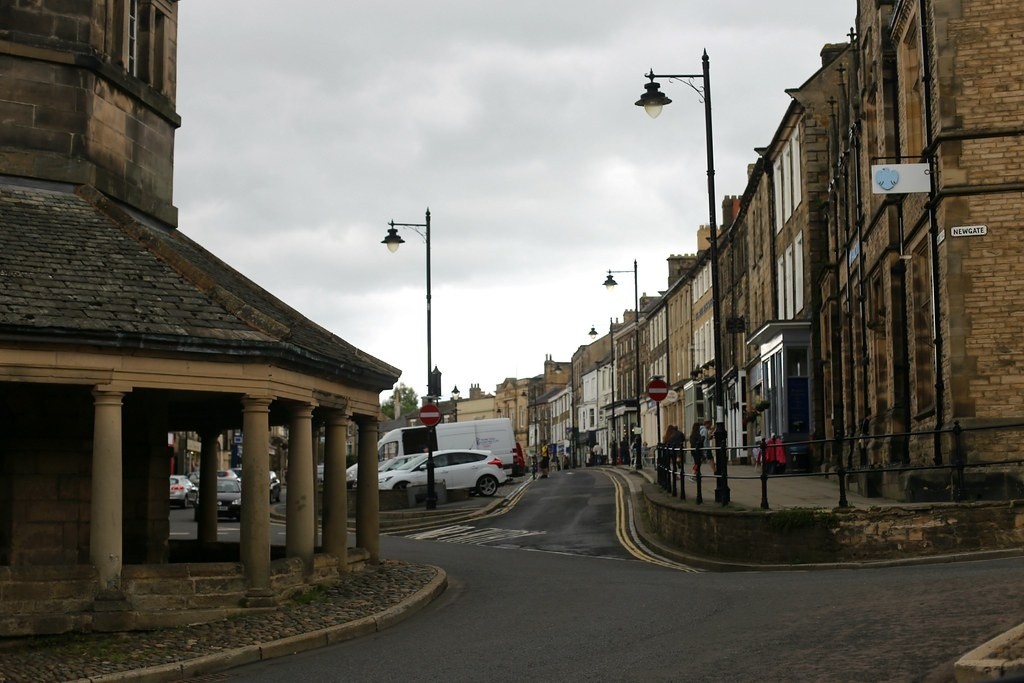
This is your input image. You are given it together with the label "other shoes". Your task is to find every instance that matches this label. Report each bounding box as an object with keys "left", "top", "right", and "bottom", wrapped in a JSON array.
[{"left": 689, "top": 477, "right": 696, "bottom": 482}]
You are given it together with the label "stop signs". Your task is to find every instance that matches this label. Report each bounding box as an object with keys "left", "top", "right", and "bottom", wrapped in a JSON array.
[
  {"left": 419, "top": 405, "right": 441, "bottom": 426},
  {"left": 647, "top": 379, "right": 668, "bottom": 401}
]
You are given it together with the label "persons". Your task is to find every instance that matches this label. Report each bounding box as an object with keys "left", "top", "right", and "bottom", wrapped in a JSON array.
[
  {"left": 688, "top": 419, "right": 718, "bottom": 483},
  {"left": 662, "top": 424, "right": 685, "bottom": 480},
  {"left": 592, "top": 437, "right": 638, "bottom": 466},
  {"left": 537, "top": 440, "right": 550, "bottom": 480}
]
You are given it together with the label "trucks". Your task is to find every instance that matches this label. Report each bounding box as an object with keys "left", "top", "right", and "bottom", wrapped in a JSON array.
[{"left": 346, "top": 418, "right": 525, "bottom": 489}]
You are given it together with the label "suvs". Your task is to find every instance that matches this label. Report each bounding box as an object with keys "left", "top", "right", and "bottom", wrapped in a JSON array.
[
  {"left": 189, "top": 470, "right": 238, "bottom": 478},
  {"left": 169, "top": 475, "right": 199, "bottom": 508}
]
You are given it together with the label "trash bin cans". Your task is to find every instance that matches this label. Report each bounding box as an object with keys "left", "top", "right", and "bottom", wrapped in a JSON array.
[{"left": 785, "top": 442, "right": 809, "bottom": 474}]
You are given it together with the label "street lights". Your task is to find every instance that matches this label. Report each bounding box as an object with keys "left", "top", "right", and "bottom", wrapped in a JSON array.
[
  {"left": 635, "top": 48, "right": 730, "bottom": 500},
  {"left": 603, "top": 262, "right": 642, "bottom": 469},
  {"left": 380, "top": 206, "right": 442, "bottom": 510},
  {"left": 589, "top": 317, "right": 617, "bottom": 466},
  {"left": 554, "top": 358, "right": 575, "bottom": 468},
  {"left": 452, "top": 386, "right": 460, "bottom": 422},
  {"left": 521, "top": 383, "right": 537, "bottom": 454}
]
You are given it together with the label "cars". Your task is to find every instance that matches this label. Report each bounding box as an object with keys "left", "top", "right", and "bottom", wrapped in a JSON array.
[
  {"left": 353, "top": 449, "right": 507, "bottom": 497},
  {"left": 230, "top": 468, "right": 281, "bottom": 505},
  {"left": 187, "top": 479, "right": 241, "bottom": 521}
]
